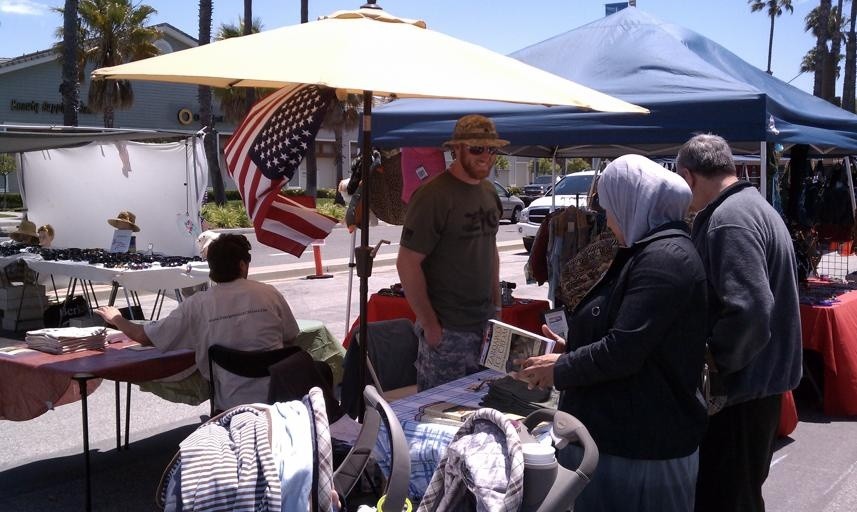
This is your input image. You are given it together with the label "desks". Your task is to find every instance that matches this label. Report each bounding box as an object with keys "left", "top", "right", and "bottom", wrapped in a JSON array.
[
  {"left": 374, "top": 368, "right": 559, "bottom": 503},
  {"left": 0, "top": 320, "right": 347, "bottom": 511},
  {"left": 796, "top": 287, "right": 857, "bottom": 420},
  {"left": 0, "top": 246, "right": 210, "bottom": 334},
  {"left": 342, "top": 292, "right": 549, "bottom": 352}
]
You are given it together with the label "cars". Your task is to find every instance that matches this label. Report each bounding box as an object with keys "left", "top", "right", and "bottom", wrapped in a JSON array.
[{"left": 492, "top": 180, "right": 525, "bottom": 224}]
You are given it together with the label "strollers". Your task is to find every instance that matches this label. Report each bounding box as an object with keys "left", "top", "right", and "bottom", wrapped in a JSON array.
[{"left": 156, "top": 386, "right": 600, "bottom": 511}]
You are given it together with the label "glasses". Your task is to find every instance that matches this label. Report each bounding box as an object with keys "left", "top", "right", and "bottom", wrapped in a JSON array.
[{"left": 465, "top": 146, "right": 499, "bottom": 156}]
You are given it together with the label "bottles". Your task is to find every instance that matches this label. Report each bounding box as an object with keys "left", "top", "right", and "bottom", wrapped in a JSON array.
[{"left": 521, "top": 442, "right": 556, "bottom": 466}]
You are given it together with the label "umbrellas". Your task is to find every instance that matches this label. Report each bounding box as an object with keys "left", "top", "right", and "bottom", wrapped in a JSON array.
[{"left": 89, "top": 0, "right": 652, "bottom": 425}]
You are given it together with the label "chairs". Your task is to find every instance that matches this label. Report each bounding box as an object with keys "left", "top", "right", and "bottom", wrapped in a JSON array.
[
  {"left": 202, "top": 344, "right": 303, "bottom": 423},
  {"left": 356, "top": 318, "right": 420, "bottom": 403},
  {"left": 268, "top": 350, "right": 379, "bottom": 501}
]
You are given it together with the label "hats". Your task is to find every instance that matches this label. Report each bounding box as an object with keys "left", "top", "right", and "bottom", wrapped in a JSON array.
[
  {"left": 442, "top": 115, "right": 511, "bottom": 148},
  {"left": 108, "top": 210, "right": 140, "bottom": 232},
  {"left": 8, "top": 220, "right": 40, "bottom": 242}
]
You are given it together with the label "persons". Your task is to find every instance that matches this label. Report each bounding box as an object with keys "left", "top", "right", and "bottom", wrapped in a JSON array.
[
  {"left": 37, "top": 224, "right": 54, "bottom": 249},
  {"left": 95, "top": 233, "right": 300, "bottom": 416},
  {"left": 512, "top": 151, "right": 709, "bottom": 511},
  {"left": 673, "top": 133, "right": 803, "bottom": 512},
  {"left": 395, "top": 114, "right": 504, "bottom": 393}
]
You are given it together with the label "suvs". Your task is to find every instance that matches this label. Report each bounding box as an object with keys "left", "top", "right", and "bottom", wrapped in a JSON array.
[
  {"left": 516, "top": 170, "right": 604, "bottom": 254},
  {"left": 518, "top": 175, "right": 561, "bottom": 203}
]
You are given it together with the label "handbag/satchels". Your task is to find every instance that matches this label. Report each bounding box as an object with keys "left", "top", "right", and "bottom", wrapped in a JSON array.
[
  {"left": 43, "top": 295, "right": 88, "bottom": 328},
  {"left": 108, "top": 306, "right": 144, "bottom": 330},
  {"left": 694, "top": 337, "right": 728, "bottom": 417}
]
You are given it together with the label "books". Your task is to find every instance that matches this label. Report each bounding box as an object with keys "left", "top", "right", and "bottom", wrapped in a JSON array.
[
  {"left": 477, "top": 320, "right": 556, "bottom": 384},
  {"left": 414, "top": 402, "right": 479, "bottom": 428}
]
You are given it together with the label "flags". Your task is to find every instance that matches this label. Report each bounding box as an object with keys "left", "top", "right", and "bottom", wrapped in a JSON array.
[{"left": 223, "top": 83, "right": 341, "bottom": 258}]
